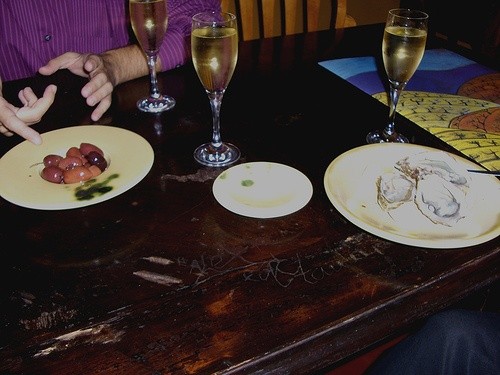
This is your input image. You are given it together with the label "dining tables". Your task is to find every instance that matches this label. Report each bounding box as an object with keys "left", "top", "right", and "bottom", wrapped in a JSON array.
[{"left": 0, "top": 22, "right": 500, "bottom": 375}]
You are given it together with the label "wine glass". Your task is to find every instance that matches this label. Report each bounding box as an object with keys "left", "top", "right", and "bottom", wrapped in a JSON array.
[
  {"left": 366, "top": 7, "right": 429, "bottom": 144},
  {"left": 129, "top": 0, "right": 176, "bottom": 113},
  {"left": 191, "top": 12, "right": 241, "bottom": 167}
]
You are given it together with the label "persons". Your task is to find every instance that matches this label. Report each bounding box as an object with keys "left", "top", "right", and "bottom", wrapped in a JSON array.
[{"left": 0, "top": 0, "right": 223, "bottom": 146}]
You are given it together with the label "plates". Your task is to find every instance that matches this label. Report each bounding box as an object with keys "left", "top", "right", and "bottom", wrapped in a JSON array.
[
  {"left": 323, "top": 143, "right": 500, "bottom": 249},
  {"left": 0, "top": 125, "right": 154, "bottom": 210}
]
